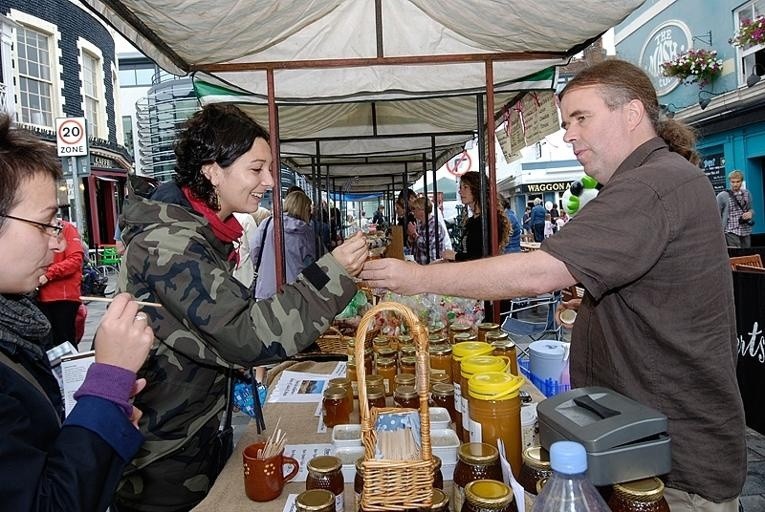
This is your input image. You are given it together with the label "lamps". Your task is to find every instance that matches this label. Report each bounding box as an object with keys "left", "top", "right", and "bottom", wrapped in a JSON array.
[
  {"left": 747, "top": 63, "right": 764, "bottom": 87},
  {"left": 662, "top": 102, "right": 696, "bottom": 119},
  {"left": 698, "top": 88, "right": 735, "bottom": 110}
]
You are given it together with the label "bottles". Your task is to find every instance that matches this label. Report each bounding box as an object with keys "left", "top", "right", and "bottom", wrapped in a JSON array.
[{"left": 536, "top": 440, "right": 613, "bottom": 512}]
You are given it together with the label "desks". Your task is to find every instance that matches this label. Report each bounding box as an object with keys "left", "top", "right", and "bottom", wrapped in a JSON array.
[{"left": 187, "top": 359, "right": 549, "bottom": 512}]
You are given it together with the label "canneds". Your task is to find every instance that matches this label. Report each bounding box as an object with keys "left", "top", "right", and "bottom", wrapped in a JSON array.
[
  {"left": 608, "top": 476, "right": 671, "bottom": 512},
  {"left": 321, "top": 322, "right": 517, "bottom": 429},
  {"left": 295, "top": 455, "right": 365, "bottom": 512},
  {"left": 428, "top": 441, "right": 551, "bottom": 512}
]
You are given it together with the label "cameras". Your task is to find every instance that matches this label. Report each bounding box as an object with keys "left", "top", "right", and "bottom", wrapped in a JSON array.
[{"left": 738, "top": 216, "right": 755, "bottom": 226}]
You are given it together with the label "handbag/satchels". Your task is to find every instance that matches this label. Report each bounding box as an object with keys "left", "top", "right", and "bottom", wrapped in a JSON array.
[{"left": 248, "top": 280, "right": 256, "bottom": 298}]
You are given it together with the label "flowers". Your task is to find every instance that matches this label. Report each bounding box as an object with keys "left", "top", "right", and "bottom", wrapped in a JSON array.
[
  {"left": 659, "top": 49, "right": 724, "bottom": 89},
  {"left": 727, "top": 15, "right": 765, "bottom": 48}
]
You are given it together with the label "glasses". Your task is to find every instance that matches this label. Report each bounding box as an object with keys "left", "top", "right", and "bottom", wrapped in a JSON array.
[{"left": 1, "top": 210, "right": 64, "bottom": 237}]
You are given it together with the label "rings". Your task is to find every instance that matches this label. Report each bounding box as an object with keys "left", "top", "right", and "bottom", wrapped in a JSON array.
[{"left": 134, "top": 314, "right": 149, "bottom": 320}]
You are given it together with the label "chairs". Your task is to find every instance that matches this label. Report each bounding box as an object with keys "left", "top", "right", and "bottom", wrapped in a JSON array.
[
  {"left": 499, "top": 288, "right": 564, "bottom": 378},
  {"left": 729, "top": 254, "right": 765, "bottom": 273},
  {"left": 560, "top": 285, "right": 585, "bottom": 302}
]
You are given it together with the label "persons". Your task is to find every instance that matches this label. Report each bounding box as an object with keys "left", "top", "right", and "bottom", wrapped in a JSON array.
[
  {"left": 34, "top": 217, "right": 84, "bottom": 354},
  {"left": 498, "top": 193, "right": 522, "bottom": 253},
  {"left": 369, "top": 187, "right": 454, "bottom": 266},
  {"left": 112, "top": 215, "right": 127, "bottom": 258},
  {"left": 521, "top": 198, "right": 569, "bottom": 242},
  {"left": 427, "top": 171, "right": 518, "bottom": 327},
  {"left": 718, "top": 169, "right": 756, "bottom": 249},
  {"left": 1, "top": 112, "right": 156, "bottom": 511},
  {"left": 363, "top": 60, "right": 748, "bottom": 511},
  {"left": 118, "top": 103, "right": 367, "bottom": 511},
  {"left": 232, "top": 186, "right": 356, "bottom": 302}
]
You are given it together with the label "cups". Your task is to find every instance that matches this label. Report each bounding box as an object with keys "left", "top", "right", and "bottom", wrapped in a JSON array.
[{"left": 242, "top": 443, "right": 298, "bottom": 502}]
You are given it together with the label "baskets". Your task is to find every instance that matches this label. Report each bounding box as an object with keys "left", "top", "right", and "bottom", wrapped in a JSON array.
[
  {"left": 356, "top": 302, "right": 441, "bottom": 511},
  {"left": 315, "top": 318, "right": 378, "bottom": 353}
]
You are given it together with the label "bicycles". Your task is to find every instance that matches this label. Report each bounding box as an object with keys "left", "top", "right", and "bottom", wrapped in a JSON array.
[{"left": 91, "top": 262, "right": 121, "bottom": 298}]
